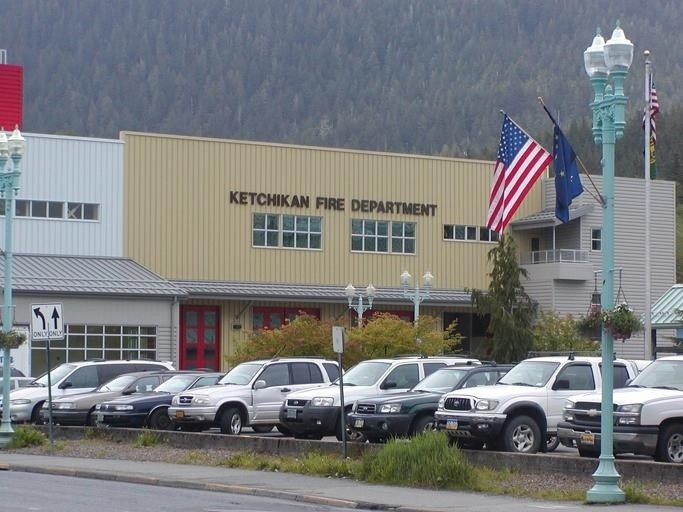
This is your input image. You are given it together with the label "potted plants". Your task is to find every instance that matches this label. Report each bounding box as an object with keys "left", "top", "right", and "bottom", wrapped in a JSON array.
[
  {"left": 603, "top": 305, "right": 643, "bottom": 340},
  {"left": 0, "top": 330, "right": 6, "bottom": 348},
  {"left": 576, "top": 311, "right": 604, "bottom": 341},
  {"left": 5, "top": 330, "right": 26, "bottom": 348}
]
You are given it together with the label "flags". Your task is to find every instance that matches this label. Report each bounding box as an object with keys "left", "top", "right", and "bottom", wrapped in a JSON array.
[
  {"left": 643, "top": 74, "right": 660, "bottom": 180},
  {"left": 486, "top": 115, "right": 553, "bottom": 237},
  {"left": 553, "top": 125, "right": 584, "bottom": 223}
]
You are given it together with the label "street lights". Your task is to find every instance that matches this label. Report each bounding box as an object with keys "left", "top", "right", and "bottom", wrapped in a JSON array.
[
  {"left": 345, "top": 280, "right": 377, "bottom": 333},
  {"left": 582, "top": 21, "right": 634, "bottom": 467},
  {"left": 1, "top": 123, "right": 28, "bottom": 435},
  {"left": 400, "top": 271, "right": 434, "bottom": 354}
]
